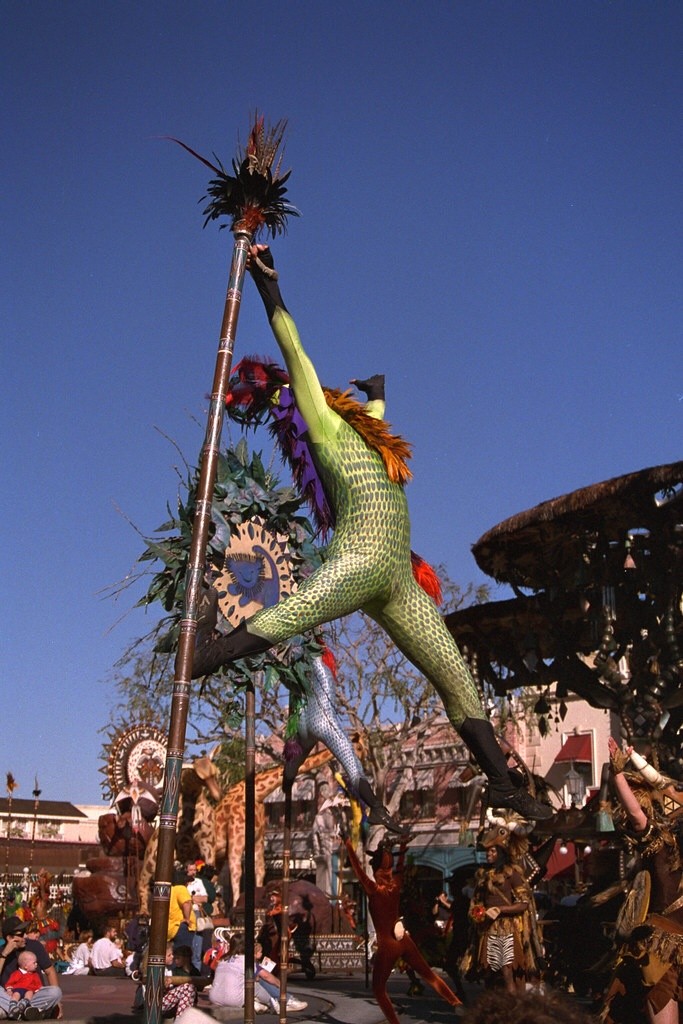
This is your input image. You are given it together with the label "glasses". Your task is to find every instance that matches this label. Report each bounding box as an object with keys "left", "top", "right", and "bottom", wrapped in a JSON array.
[{"left": 10, "top": 928, "right": 26, "bottom": 938}]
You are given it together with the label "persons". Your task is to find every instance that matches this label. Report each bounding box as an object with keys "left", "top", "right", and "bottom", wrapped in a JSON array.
[
  {"left": 261, "top": 630, "right": 402, "bottom": 839},
  {"left": 179, "top": 240, "right": 559, "bottom": 822},
  {"left": 0, "top": 736, "right": 683, "bottom": 1024}
]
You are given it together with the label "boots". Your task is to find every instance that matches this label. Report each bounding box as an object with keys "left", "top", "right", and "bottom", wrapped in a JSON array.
[
  {"left": 357, "top": 777, "right": 406, "bottom": 835},
  {"left": 173, "top": 589, "right": 275, "bottom": 679},
  {"left": 282, "top": 757, "right": 306, "bottom": 793},
  {"left": 456, "top": 716, "right": 555, "bottom": 819}
]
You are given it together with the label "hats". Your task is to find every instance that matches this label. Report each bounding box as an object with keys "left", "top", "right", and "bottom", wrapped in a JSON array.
[
  {"left": 2, "top": 916, "right": 31, "bottom": 936},
  {"left": 172, "top": 870, "right": 195, "bottom": 885}
]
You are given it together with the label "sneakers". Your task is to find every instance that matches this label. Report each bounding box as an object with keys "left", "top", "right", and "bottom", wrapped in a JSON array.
[{"left": 286, "top": 995, "right": 308, "bottom": 1011}]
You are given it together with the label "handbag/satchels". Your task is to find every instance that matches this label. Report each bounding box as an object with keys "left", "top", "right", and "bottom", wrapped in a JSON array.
[{"left": 195, "top": 904, "right": 215, "bottom": 932}]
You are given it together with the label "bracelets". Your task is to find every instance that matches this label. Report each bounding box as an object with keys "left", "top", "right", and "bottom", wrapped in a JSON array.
[{"left": 0, "top": 953, "right": 6, "bottom": 959}]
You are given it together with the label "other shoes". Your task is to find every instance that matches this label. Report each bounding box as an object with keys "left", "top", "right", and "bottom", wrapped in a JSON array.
[
  {"left": 254, "top": 1001, "right": 268, "bottom": 1015},
  {"left": 8, "top": 999, "right": 28, "bottom": 1015},
  {"left": 24, "top": 1005, "right": 47, "bottom": 1020},
  {"left": 269, "top": 998, "right": 280, "bottom": 1015}
]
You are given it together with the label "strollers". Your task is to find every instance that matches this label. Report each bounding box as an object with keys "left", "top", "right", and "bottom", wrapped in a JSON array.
[{"left": 125, "top": 913, "right": 152, "bottom": 982}]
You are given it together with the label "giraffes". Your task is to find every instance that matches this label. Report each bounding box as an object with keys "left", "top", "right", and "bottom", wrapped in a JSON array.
[{"left": 136, "top": 733, "right": 369, "bottom": 925}]
